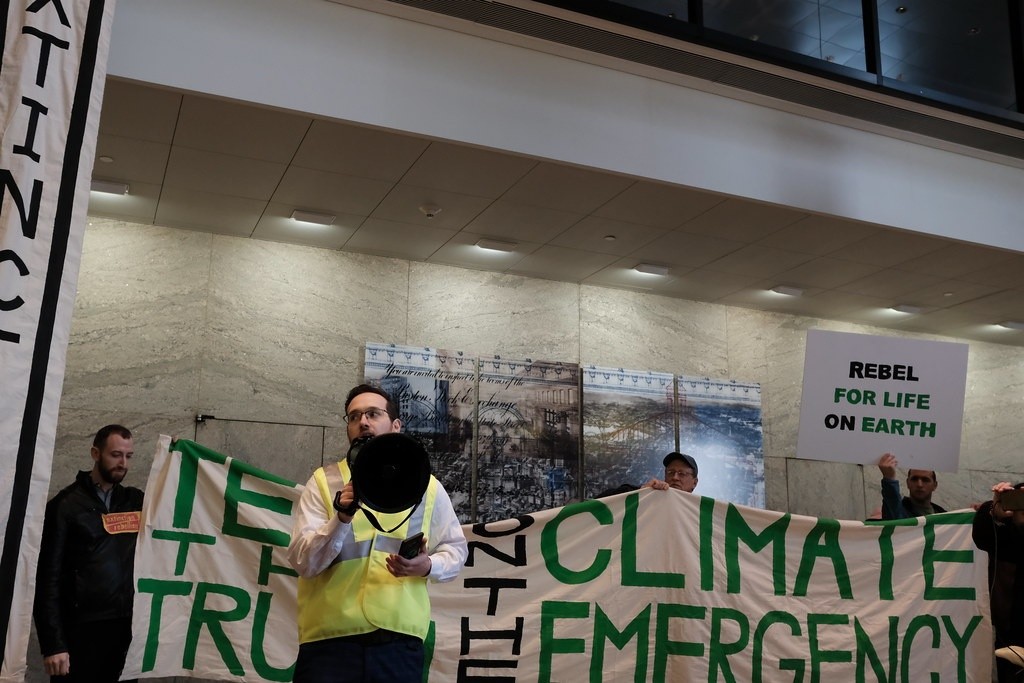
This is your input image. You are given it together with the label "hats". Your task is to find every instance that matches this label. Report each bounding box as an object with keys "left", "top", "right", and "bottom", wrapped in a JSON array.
[{"left": 663, "top": 450, "right": 697, "bottom": 477}]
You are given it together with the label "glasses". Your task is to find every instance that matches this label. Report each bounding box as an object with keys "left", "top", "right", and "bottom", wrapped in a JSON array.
[
  {"left": 665, "top": 469, "right": 694, "bottom": 477},
  {"left": 342, "top": 409, "right": 395, "bottom": 425}
]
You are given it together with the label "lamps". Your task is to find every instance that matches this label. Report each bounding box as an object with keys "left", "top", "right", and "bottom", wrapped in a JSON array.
[
  {"left": 890, "top": 304, "right": 921, "bottom": 314},
  {"left": 632, "top": 263, "right": 670, "bottom": 277},
  {"left": 770, "top": 285, "right": 805, "bottom": 297},
  {"left": 473, "top": 239, "right": 521, "bottom": 252},
  {"left": 999, "top": 320, "right": 1024, "bottom": 329},
  {"left": 90, "top": 180, "right": 129, "bottom": 195},
  {"left": 289, "top": 209, "right": 338, "bottom": 226}
]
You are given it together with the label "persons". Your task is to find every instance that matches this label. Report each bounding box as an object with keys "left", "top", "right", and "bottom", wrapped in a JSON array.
[
  {"left": 969, "top": 481, "right": 1024, "bottom": 683},
  {"left": 33, "top": 424, "right": 180, "bottom": 683},
  {"left": 878, "top": 453, "right": 947, "bottom": 520},
  {"left": 285, "top": 382, "right": 468, "bottom": 683},
  {"left": 593, "top": 451, "right": 699, "bottom": 499}
]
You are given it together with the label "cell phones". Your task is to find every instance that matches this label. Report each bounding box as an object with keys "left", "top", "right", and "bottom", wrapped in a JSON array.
[
  {"left": 1000, "top": 489, "right": 1024, "bottom": 512},
  {"left": 397, "top": 532, "right": 424, "bottom": 560}
]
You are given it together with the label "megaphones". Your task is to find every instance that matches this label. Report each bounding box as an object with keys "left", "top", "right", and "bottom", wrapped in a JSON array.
[{"left": 347, "top": 432, "right": 431, "bottom": 514}]
